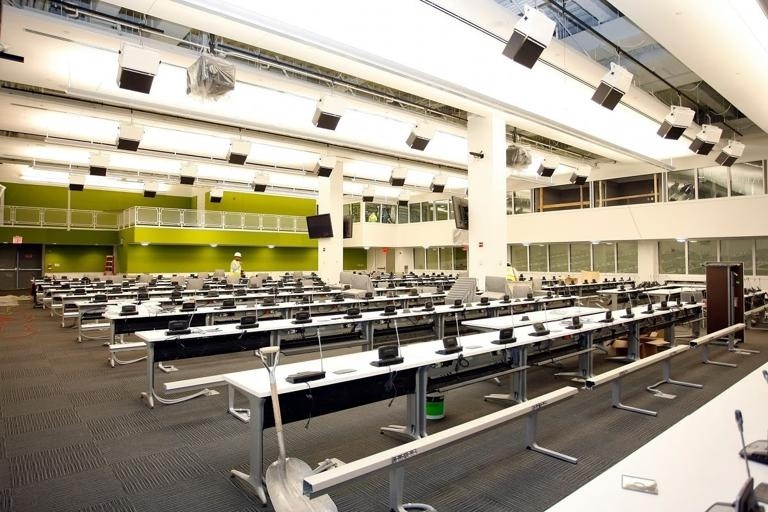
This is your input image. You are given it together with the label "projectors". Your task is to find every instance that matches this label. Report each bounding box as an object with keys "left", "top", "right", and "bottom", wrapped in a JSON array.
[
  {"left": 185, "top": 54, "right": 236, "bottom": 97},
  {"left": 506, "top": 145, "right": 532, "bottom": 166}
]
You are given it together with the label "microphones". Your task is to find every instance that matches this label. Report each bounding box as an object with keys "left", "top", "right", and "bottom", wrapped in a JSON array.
[
  {"left": 286, "top": 327, "right": 325, "bottom": 384},
  {"left": 262, "top": 271, "right": 345, "bottom": 324},
  {"left": 352, "top": 269, "right": 512, "bottom": 316},
  {"left": 705, "top": 410, "right": 765, "bottom": 512},
  {"left": 738, "top": 370, "right": 768, "bottom": 466},
  {"left": 344, "top": 294, "right": 362, "bottom": 319},
  {"left": 491, "top": 308, "right": 516, "bottom": 345},
  {"left": 369, "top": 319, "right": 404, "bottom": 367},
  {"left": 743, "top": 277, "right": 762, "bottom": 296},
  {"left": 37, "top": 274, "right": 112, "bottom": 318},
  {"left": 165, "top": 302, "right": 198, "bottom": 335},
  {"left": 435, "top": 313, "right": 463, "bottom": 355},
  {"left": 519, "top": 271, "right": 707, "bottom": 337},
  {"left": 234, "top": 300, "right": 260, "bottom": 330},
  {"left": 112, "top": 271, "right": 251, "bottom": 316}
]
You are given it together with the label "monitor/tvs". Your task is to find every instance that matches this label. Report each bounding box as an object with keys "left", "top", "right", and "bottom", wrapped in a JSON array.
[
  {"left": 306, "top": 213, "right": 333, "bottom": 239},
  {"left": 452, "top": 196, "right": 468, "bottom": 230},
  {"left": 343, "top": 215, "right": 352, "bottom": 238}
]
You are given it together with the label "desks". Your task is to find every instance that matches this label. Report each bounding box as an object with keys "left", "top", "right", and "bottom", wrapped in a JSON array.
[
  {"left": 222, "top": 270, "right": 768, "bottom": 512},
  {"left": 29, "top": 272, "right": 303, "bottom": 409}
]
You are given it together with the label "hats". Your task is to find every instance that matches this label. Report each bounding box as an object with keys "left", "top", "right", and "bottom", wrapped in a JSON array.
[{"left": 234, "top": 252, "right": 242, "bottom": 257}]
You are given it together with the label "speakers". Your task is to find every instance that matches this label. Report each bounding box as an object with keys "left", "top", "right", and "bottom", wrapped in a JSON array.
[
  {"left": 69, "top": 174, "right": 86, "bottom": 191},
  {"left": 143, "top": 141, "right": 267, "bottom": 203},
  {"left": 117, "top": 122, "right": 145, "bottom": 152},
  {"left": 656, "top": 104, "right": 746, "bottom": 167},
  {"left": 312, "top": 160, "right": 334, "bottom": 178},
  {"left": 89, "top": 153, "right": 112, "bottom": 176},
  {"left": 311, "top": 106, "right": 342, "bottom": 131},
  {"left": 363, "top": 167, "right": 445, "bottom": 207},
  {"left": 536, "top": 157, "right": 589, "bottom": 185},
  {"left": 406, "top": 130, "right": 431, "bottom": 152}
]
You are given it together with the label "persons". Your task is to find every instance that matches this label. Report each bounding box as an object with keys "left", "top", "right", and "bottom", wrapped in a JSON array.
[
  {"left": 230, "top": 251, "right": 242, "bottom": 277},
  {"left": 507, "top": 261, "right": 517, "bottom": 282},
  {"left": 368, "top": 212, "right": 377, "bottom": 222}
]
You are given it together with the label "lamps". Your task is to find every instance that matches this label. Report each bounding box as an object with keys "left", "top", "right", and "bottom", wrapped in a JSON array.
[
  {"left": 225, "top": 126, "right": 249, "bottom": 165},
  {"left": 251, "top": 167, "right": 269, "bottom": 192},
  {"left": 657, "top": 91, "right": 695, "bottom": 141},
  {"left": 690, "top": 112, "right": 724, "bottom": 156},
  {"left": 398, "top": 194, "right": 409, "bottom": 207},
  {"left": 68, "top": 167, "right": 85, "bottom": 192},
  {"left": 429, "top": 176, "right": 446, "bottom": 193},
  {"left": 313, "top": 96, "right": 341, "bottom": 130},
  {"left": 570, "top": 161, "right": 589, "bottom": 185},
  {"left": 209, "top": 180, "right": 223, "bottom": 203},
  {"left": 115, "top": 109, "right": 146, "bottom": 152},
  {"left": 405, "top": 125, "right": 431, "bottom": 151},
  {"left": 143, "top": 177, "right": 157, "bottom": 198},
  {"left": 362, "top": 189, "right": 375, "bottom": 202},
  {"left": 715, "top": 130, "right": 744, "bottom": 167},
  {"left": 537, "top": 151, "right": 558, "bottom": 177},
  {"left": 186, "top": 39, "right": 235, "bottom": 101},
  {"left": 388, "top": 170, "right": 406, "bottom": 187},
  {"left": 314, "top": 157, "right": 335, "bottom": 178},
  {"left": 505, "top": 127, "right": 530, "bottom": 167},
  {"left": 180, "top": 159, "right": 196, "bottom": 184},
  {"left": 88, "top": 144, "right": 108, "bottom": 177}
]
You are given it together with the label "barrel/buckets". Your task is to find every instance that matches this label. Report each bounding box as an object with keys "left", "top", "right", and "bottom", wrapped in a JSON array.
[{"left": 425, "top": 391, "right": 445, "bottom": 420}]
